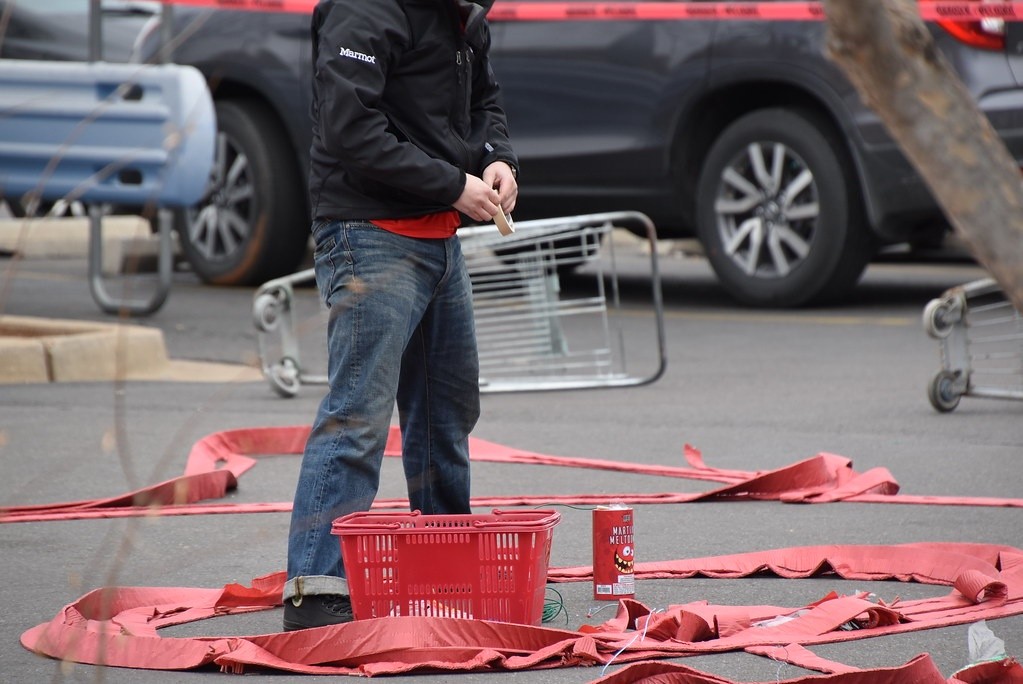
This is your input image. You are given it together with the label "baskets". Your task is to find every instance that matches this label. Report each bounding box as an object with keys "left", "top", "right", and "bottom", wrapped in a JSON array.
[{"left": 331, "top": 509, "right": 561, "bottom": 629}]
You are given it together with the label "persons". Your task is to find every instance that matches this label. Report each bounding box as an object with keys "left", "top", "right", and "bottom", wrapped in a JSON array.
[{"left": 281, "top": 0, "right": 522, "bottom": 635}]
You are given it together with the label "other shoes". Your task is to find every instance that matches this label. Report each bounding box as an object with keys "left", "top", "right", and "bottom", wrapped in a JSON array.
[{"left": 282, "top": 593, "right": 359, "bottom": 630}]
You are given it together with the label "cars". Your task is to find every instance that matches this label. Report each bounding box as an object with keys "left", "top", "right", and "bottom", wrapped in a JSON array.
[{"left": 120, "top": 2, "right": 1023, "bottom": 314}]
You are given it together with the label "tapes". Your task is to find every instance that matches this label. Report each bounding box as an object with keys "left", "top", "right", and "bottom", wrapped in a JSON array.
[{"left": 491, "top": 188, "right": 516, "bottom": 236}]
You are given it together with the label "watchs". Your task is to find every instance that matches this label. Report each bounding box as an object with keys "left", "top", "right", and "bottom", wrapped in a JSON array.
[{"left": 504, "top": 161, "right": 517, "bottom": 179}]
